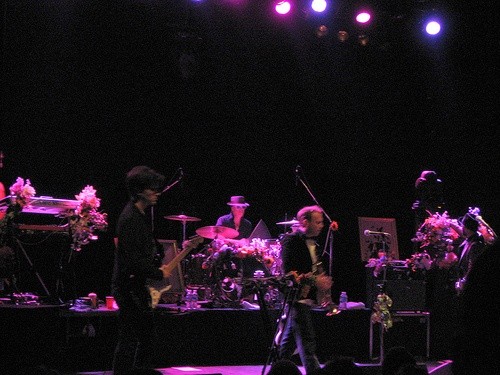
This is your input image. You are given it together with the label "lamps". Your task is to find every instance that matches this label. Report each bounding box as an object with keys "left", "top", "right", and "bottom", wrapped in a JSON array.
[
  {"left": 318, "top": 24, "right": 328, "bottom": 33},
  {"left": 358, "top": 33, "right": 369, "bottom": 45},
  {"left": 337, "top": 31, "right": 348, "bottom": 42}
]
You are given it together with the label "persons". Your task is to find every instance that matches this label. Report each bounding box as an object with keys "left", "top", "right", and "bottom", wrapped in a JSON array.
[
  {"left": 453, "top": 213, "right": 485, "bottom": 363},
  {"left": 279, "top": 205, "right": 334, "bottom": 375},
  {"left": 216, "top": 196, "right": 252, "bottom": 241},
  {"left": 111, "top": 166, "right": 172, "bottom": 375}
]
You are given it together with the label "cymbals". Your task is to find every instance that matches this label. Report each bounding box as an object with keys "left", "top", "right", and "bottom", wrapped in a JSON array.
[
  {"left": 196, "top": 226, "right": 239, "bottom": 240},
  {"left": 164, "top": 215, "right": 202, "bottom": 222},
  {"left": 276, "top": 221, "right": 299, "bottom": 225}
]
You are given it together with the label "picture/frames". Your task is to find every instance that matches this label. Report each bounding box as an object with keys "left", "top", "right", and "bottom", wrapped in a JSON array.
[
  {"left": 359, "top": 217, "right": 399, "bottom": 261},
  {"left": 114, "top": 237, "right": 186, "bottom": 295}
]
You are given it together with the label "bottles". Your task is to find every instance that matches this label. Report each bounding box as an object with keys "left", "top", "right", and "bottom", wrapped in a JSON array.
[
  {"left": 185, "top": 290, "right": 192, "bottom": 309},
  {"left": 191, "top": 290, "right": 198, "bottom": 309},
  {"left": 340, "top": 292, "right": 347, "bottom": 310}
]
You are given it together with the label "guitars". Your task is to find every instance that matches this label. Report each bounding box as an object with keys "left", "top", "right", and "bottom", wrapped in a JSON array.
[{"left": 143, "top": 234, "right": 204, "bottom": 308}]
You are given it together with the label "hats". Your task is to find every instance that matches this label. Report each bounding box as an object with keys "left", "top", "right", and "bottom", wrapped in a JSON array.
[{"left": 227, "top": 196, "right": 250, "bottom": 206}]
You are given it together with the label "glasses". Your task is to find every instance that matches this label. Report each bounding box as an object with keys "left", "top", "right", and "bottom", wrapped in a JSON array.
[{"left": 235, "top": 206, "right": 246, "bottom": 210}]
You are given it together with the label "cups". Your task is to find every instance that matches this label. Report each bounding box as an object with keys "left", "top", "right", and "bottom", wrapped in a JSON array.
[{"left": 106, "top": 297, "right": 114, "bottom": 309}]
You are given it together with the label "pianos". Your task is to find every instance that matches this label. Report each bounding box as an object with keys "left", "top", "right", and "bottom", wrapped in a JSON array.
[{"left": 0, "top": 196, "right": 92, "bottom": 230}]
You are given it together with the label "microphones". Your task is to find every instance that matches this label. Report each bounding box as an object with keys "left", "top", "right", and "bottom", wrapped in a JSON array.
[
  {"left": 295, "top": 166, "right": 299, "bottom": 186},
  {"left": 180, "top": 169, "right": 184, "bottom": 188},
  {"left": 364, "top": 230, "right": 391, "bottom": 237}
]
